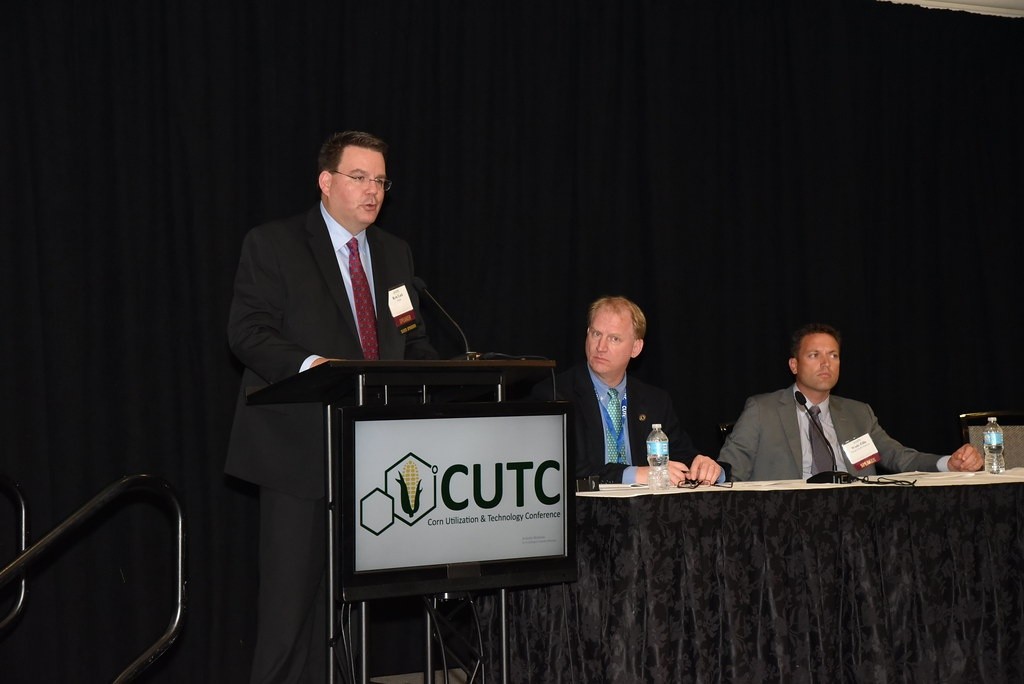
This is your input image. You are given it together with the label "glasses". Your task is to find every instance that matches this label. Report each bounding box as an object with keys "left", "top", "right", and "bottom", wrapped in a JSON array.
[{"left": 329, "top": 170, "right": 392, "bottom": 190}]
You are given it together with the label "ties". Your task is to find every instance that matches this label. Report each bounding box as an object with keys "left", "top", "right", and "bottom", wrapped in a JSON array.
[
  {"left": 806, "top": 406, "right": 834, "bottom": 476},
  {"left": 605, "top": 388, "right": 629, "bottom": 465},
  {"left": 346, "top": 237, "right": 379, "bottom": 360}
]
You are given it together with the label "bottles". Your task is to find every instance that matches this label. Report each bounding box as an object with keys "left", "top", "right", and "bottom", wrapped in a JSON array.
[
  {"left": 646, "top": 423, "right": 673, "bottom": 492},
  {"left": 983, "top": 416, "right": 1006, "bottom": 474}
]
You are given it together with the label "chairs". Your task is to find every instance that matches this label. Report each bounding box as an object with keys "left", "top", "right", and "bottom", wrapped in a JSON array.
[{"left": 959, "top": 410, "right": 1024, "bottom": 470}]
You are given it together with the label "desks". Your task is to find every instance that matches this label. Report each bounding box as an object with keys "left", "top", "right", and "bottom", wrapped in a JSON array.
[{"left": 468, "top": 462, "right": 1024, "bottom": 683}]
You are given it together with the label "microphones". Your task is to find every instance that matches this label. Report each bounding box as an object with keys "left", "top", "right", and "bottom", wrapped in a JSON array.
[
  {"left": 411, "top": 277, "right": 483, "bottom": 360},
  {"left": 795, "top": 391, "right": 855, "bottom": 484},
  {"left": 483, "top": 352, "right": 564, "bottom": 416}
]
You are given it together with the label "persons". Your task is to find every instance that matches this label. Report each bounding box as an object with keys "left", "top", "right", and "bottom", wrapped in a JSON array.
[
  {"left": 714, "top": 323, "right": 986, "bottom": 481},
  {"left": 554, "top": 293, "right": 735, "bottom": 489},
  {"left": 222, "top": 129, "right": 441, "bottom": 682}
]
